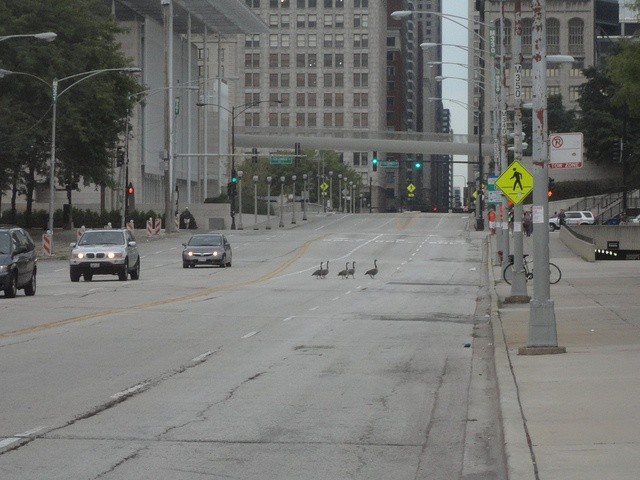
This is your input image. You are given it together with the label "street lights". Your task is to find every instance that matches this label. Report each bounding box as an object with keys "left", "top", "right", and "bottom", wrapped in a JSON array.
[
  {"left": 237, "top": 171, "right": 243, "bottom": 229},
  {"left": 344, "top": 177, "right": 347, "bottom": 213},
  {"left": 353, "top": 185, "right": 356, "bottom": 214},
  {"left": 253, "top": 176, "right": 260, "bottom": 230},
  {"left": 419, "top": 41, "right": 502, "bottom": 266},
  {"left": 279, "top": 176, "right": 285, "bottom": 227},
  {"left": 121, "top": 85, "right": 200, "bottom": 228},
  {"left": 359, "top": 194, "right": 363, "bottom": 212},
  {"left": 364, "top": 198, "right": 367, "bottom": 213},
  {"left": 291, "top": 175, "right": 296, "bottom": 223},
  {"left": 302, "top": 173, "right": 308, "bottom": 220},
  {"left": 390, "top": 9, "right": 510, "bottom": 280},
  {"left": 338, "top": 174, "right": 342, "bottom": 212},
  {"left": 349, "top": 182, "right": 352, "bottom": 213},
  {"left": 196, "top": 100, "right": 283, "bottom": 228},
  {"left": 329, "top": 171, "right": 333, "bottom": 212},
  {"left": 0, "top": 67, "right": 142, "bottom": 255},
  {"left": 266, "top": 176, "right": 272, "bottom": 230}
]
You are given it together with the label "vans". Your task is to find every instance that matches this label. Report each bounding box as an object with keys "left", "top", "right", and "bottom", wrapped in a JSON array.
[{"left": 548, "top": 211, "right": 595, "bottom": 232}]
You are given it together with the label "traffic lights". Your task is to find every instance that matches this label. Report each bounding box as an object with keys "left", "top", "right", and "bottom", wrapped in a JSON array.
[
  {"left": 416, "top": 153, "right": 423, "bottom": 179},
  {"left": 128, "top": 185, "right": 134, "bottom": 194},
  {"left": 373, "top": 150, "right": 377, "bottom": 172},
  {"left": 232, "top": 170, "right": 236, "bottom": 195}
]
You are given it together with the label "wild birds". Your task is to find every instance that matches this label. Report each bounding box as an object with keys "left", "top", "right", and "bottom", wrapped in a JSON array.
[
  {"left": 321, "top": 259, "right": 330, "bottom": 279},
  {"left": 311, "top": 261, "right": 326, "bottom": 281},
  {"left": 336, "top": 261, "right": 351, "bottom": 281},
  {"left": 363, "top": 258, "right": 379, "bottom": 280},
  {"left": 346, "top": 260, "right": 357, "bottom": 280}
]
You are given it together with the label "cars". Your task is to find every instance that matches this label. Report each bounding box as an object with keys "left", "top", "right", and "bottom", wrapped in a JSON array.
[{"left": 183, "top": 233, "right": 232, "bottom": 268}]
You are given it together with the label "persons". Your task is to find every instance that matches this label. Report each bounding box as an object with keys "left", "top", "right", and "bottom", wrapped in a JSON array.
[
  {"left": 508, "top": 207, "right": 514, "bottom": 231},
  {"left": 553, "top": 211, "right": 558, "bottom": 218},
  {"left": 559, "top": 209, "right": 566, "bottom": 225},
  {"left": 523, "top": 207, "right": 532, "bottom": 238},
  {"left": 488, "top": 209, "right": 496, "bottom": 234}
]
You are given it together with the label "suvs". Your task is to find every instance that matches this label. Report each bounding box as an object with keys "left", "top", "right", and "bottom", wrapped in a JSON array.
[
  {"left": 70, "top": 229, "right": 140, "bottom": 282},
  {"left": 0, "top": 227, "right": 37, "bottom": 298}
]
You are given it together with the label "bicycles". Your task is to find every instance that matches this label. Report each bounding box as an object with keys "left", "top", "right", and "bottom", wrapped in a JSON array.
[{"left": 503, "top": 254, "right": 561, "bottom": 286}]
